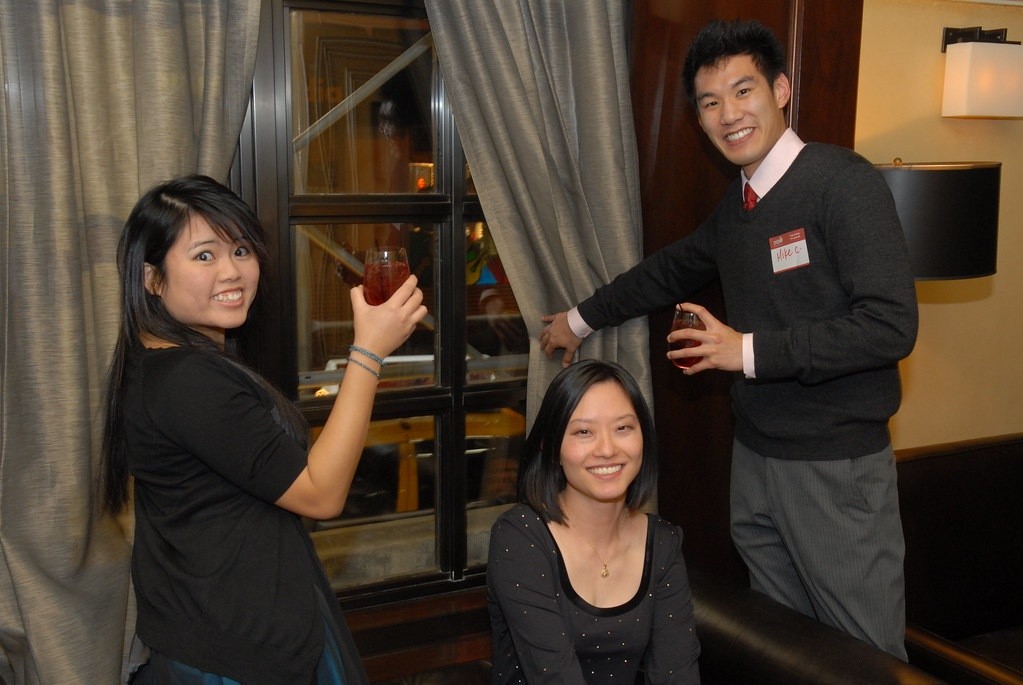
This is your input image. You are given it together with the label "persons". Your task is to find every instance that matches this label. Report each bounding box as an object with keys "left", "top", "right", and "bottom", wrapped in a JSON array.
[
  {"left": 98, "top": 175, "right": 429, "bottom": 685},
  {"left": 546, "top": 20, "right": 919, "bottom": 669},
  {"left": 485, "top": 357, "right": 703, "bottom": 685}
]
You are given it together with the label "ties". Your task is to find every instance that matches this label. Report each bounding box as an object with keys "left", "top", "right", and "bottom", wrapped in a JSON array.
[{"left": 744, "top": 182, "right": 758, "bottom": 210}]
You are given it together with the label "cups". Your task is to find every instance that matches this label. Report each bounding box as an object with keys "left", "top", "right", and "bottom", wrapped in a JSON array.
[
  {"left": 363, "top": 247, "right": 409, "bottom": 309},
  {"left": 669, "top": 313, "right": 707, "bottom": 368}
]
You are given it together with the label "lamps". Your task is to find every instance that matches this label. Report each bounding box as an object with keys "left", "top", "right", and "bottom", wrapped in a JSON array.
[{"left": 938, "top": 23, "right": 1023, "bottom": 119}]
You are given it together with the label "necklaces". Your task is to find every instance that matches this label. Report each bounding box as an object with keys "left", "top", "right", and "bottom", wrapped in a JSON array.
[{"left": 563, "top": 508, "right": 628, "bottom": 577}]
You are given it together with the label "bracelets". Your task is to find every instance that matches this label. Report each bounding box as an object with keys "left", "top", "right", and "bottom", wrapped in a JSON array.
[{"left": 348, "top": 345, "right": 386, "bottom": 378}]
